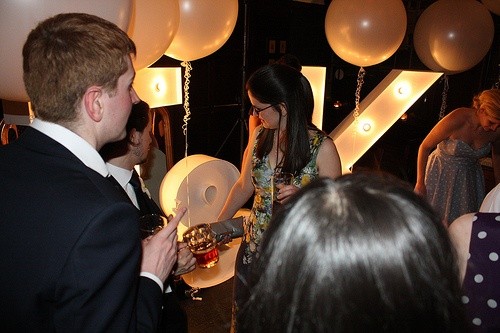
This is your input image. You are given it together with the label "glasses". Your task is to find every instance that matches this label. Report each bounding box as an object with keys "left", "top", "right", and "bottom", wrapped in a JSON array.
[{"left": 252, "top": 100, "right": 275, "bottom": 114}]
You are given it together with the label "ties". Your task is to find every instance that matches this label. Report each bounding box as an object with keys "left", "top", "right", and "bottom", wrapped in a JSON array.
[{"left": 129, "top": 171, "right": 146, "bottom": 211}]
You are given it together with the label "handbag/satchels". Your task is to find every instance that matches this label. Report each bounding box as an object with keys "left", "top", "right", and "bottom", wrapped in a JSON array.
[{"left": 210, "top": 216, "right": 245, "bottom": 241}]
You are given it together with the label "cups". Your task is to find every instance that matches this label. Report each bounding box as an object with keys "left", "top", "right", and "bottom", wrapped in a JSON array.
[
  {"left": 139, "top": 214, "right": 167, "bottom": 238},
  {"left": 185, "top": 224, "right": 220, "bottom": 267}
]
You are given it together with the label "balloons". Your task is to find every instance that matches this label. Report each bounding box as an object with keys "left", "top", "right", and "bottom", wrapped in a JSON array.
[
  {"left": 0, "top": 0, "right": 238, "bottom": 102},
  {"left": 325, "top": 0, "right": 407, "bottom": 69},
  {"left": 413, "top": 0, "right": 500, "bottom": 76}
]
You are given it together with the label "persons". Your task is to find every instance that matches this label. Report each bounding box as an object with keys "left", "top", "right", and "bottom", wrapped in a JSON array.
[
  {"left": 0, "top": 12, "right": 199, "bottom": 333},
  {"left": 214, "top": 63, "right": 341, "bottom": 333},
  {"left": 237, "top": 173, "right": 499, "bottom": 333},
  {"left": 97, "top": 99, "right": 202, "bottom": 333},
  {"left": 412, "top": 88, "right": 500, "bottom": 230}
]
